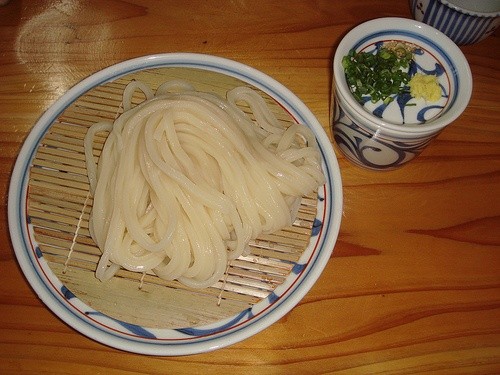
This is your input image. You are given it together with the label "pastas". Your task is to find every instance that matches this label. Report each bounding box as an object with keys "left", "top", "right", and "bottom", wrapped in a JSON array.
[{"left": 83, "top": 80, "right": 327, "bottom": 289}]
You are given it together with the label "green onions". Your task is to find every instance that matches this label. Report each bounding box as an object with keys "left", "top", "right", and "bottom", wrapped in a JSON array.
[{"left": 341, "top": 46, "right": 424, "bottom": 107}]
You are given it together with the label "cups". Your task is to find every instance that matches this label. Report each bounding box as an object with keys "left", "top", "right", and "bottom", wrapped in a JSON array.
[
  {"left": 328, "top": 75, "right": 446, "bottom": 172},
  {"left": 410, "top": 0, "right": 500, "bottom": 45}
]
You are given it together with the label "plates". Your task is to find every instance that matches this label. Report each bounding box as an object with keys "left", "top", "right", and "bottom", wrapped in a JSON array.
[
  {"left": 7, "top": 52, "right": 344, "bottom": 356},
  {"left": 333, "top": 17, "right": 473, "bottom": 133}
]
